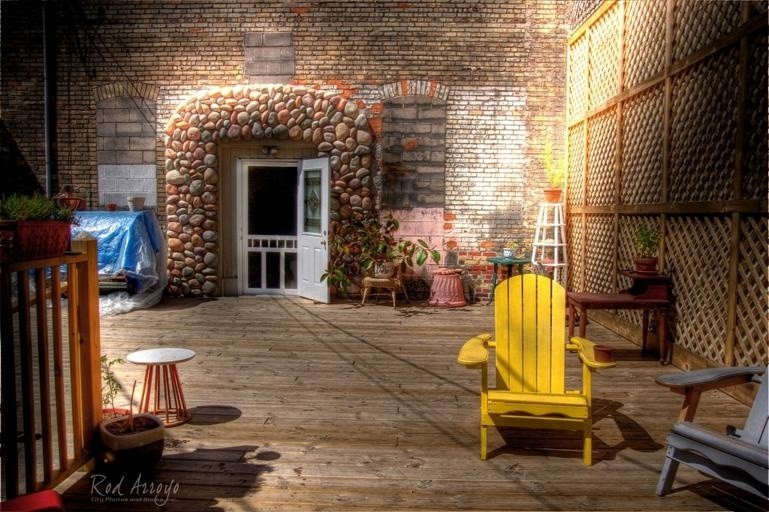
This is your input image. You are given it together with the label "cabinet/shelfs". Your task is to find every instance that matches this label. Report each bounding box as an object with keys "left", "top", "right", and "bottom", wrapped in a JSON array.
[{"left": 530, "top": 203, "right": 569, "bottom": 285}]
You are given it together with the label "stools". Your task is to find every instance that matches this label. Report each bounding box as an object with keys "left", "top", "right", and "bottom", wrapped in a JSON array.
[
  {"left": 428, "top": 268, "right": 466, "bottom": 307},
  {"left": 128, "top": 346, "right": 197, "bottom": 426}
]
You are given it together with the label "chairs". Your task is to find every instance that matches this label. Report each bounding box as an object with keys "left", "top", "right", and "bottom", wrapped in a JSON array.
[
  {"left": 654, "top": 366, "right": 768, "bottom": 512},
  {"left": 458, "top": 273, "right": 617, "bottom": 465},
  {"left": 362, "top": 263, "right": 410, "bottom": 308}
]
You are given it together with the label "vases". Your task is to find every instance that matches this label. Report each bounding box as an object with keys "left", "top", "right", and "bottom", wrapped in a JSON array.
[
  {"left": 106, "top": 203, "right": 117, "bottom": 211},
  {"left": 127, "top": 196, "right": 146, "bottom": 212},
  {"left": 55, "top": 197, "right": 80, "bottom": 211}
]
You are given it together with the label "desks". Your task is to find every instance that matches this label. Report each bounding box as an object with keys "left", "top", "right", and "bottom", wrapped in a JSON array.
[
  {"left": 487, "top": 257, "right": 531, "bottom": 305},
  {"left": 566, "top": 269, "right": 674, "bottom": 366}
]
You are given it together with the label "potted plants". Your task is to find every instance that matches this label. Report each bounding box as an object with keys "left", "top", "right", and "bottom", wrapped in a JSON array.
[
  {"left": 543, "top": 127, "right": 566, "bottom": 203},
  {"left": 319, "top": 215, "right": 441, "bottom": 289},
  {"left": 633, "top": 221, "right": 663, "bottom": 274},
  {"left": 100, "top": 381, "right": 164, "bottom": 481},
  {"left": 503, "top": 240, "right": 519, "bottom": 257},
  {"left": 0, "top": 192, "right": 77, "bottom": 258},
  {"left": 100, "top": 354, "right": 129, "bottom": 421}
]
